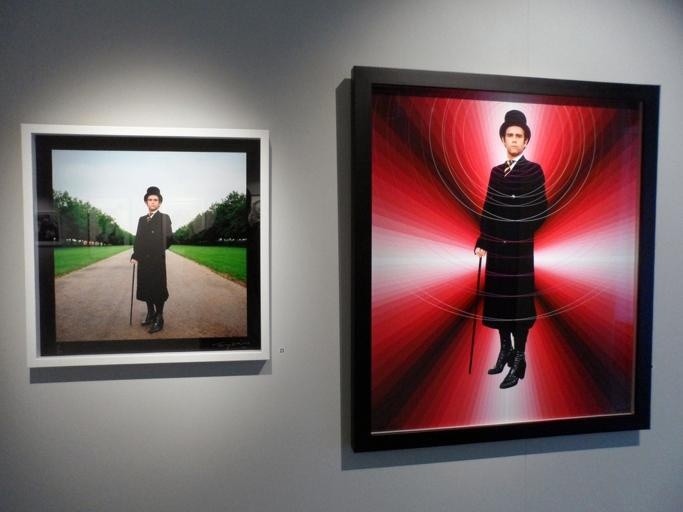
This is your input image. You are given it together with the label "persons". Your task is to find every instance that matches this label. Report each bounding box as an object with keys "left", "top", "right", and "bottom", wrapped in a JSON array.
[
  {"left": 474, "top": 109, "right": 546, "bottom": 390},
  {"left": 128, "top": 185, "right": 173, "bottom": 334}
]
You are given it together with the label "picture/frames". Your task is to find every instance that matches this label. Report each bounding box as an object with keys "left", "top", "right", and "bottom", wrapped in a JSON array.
[
  {"left": 347, "top": 64, "right": 662, "bottom": 455},
  {"left": 19, "top": 122, "right": 272, "bottom": 373}
]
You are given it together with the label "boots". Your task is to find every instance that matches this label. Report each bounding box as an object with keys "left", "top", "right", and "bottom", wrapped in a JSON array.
[
  {"left": 148, "top": 314, "right": 163, "bottom": 334},
  {"left": 487, "top": 343, "right": 514, "bottom": 374},
  {"left": 141, "top": 307, "right": 156, "bottom": 326},
  {"left": 499, "top": 352, "right": 526, "bottom": 389}
]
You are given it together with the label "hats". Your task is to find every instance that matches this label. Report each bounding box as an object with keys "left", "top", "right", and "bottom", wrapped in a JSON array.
[
  {"left": 144, "top": 186, "right": 162, "bottom": 204},
  {"left": 499, "top": 110, "right": 530, "bottom": 143}
]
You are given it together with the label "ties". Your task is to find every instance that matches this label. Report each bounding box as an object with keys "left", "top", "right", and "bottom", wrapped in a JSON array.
[{"left": 503, "top": 160, "right": 514, "bottom": 176}]
